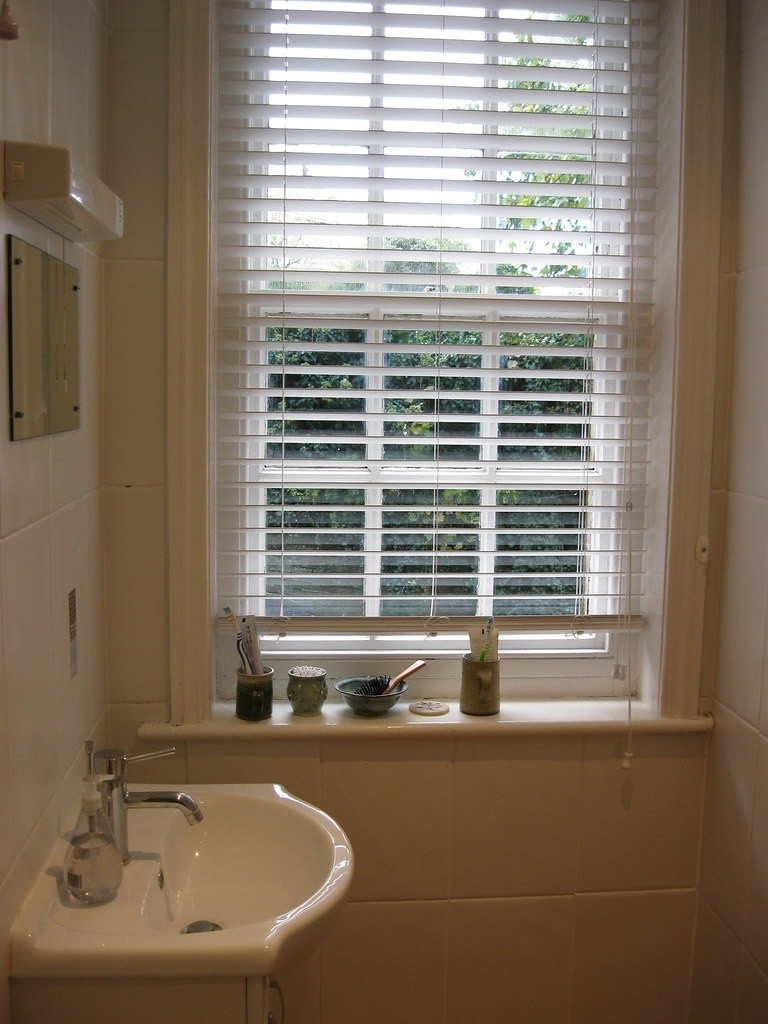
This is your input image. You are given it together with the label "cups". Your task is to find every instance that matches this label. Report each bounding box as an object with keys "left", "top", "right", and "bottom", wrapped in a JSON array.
[
  {"left": 460, "top": 652, "right": 500, "bottom": 716},
  {"left": 287, "top": 667, "right": 328, "bottom": 716},
  {"left": 236, "top": 665, "right": 274, "bottom": 721}
]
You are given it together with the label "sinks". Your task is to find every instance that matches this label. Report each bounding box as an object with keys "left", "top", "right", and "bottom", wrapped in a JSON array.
[{"left": 8, "top": 776, "right": 358, "bottom": 984}]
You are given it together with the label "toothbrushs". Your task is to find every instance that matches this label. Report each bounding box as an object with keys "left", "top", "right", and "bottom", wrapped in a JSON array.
[
  {"left": 477, "top": 615, "right": 497, "bottom": 664},
  {"left": 221, "top": 605, "right": 253, "bottom": 676}
]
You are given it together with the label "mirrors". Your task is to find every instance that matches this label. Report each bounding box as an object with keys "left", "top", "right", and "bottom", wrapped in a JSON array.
[{"left": 7, "top": 233, "right": 81, "bottom": 441}]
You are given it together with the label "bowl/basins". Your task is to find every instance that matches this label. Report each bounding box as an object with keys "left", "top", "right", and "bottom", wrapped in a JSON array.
[{"left": 333, "top": 676, "right": 409, "bottom": 717}]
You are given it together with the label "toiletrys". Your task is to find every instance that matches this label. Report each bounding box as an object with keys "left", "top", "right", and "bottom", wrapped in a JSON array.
[
  {"left": 468, "top": 626, "right": 502, "bottom": 662},
  {"left": 65, "top": 772, "right": 127, "bottom": 906},
  {"left": 235, "top": 613, "right": 267, "bottom": 676}
]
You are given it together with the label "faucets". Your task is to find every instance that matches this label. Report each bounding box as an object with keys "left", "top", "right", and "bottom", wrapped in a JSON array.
[{"left": 89, "top": 745, "right": 206, "bottom": 865}]
